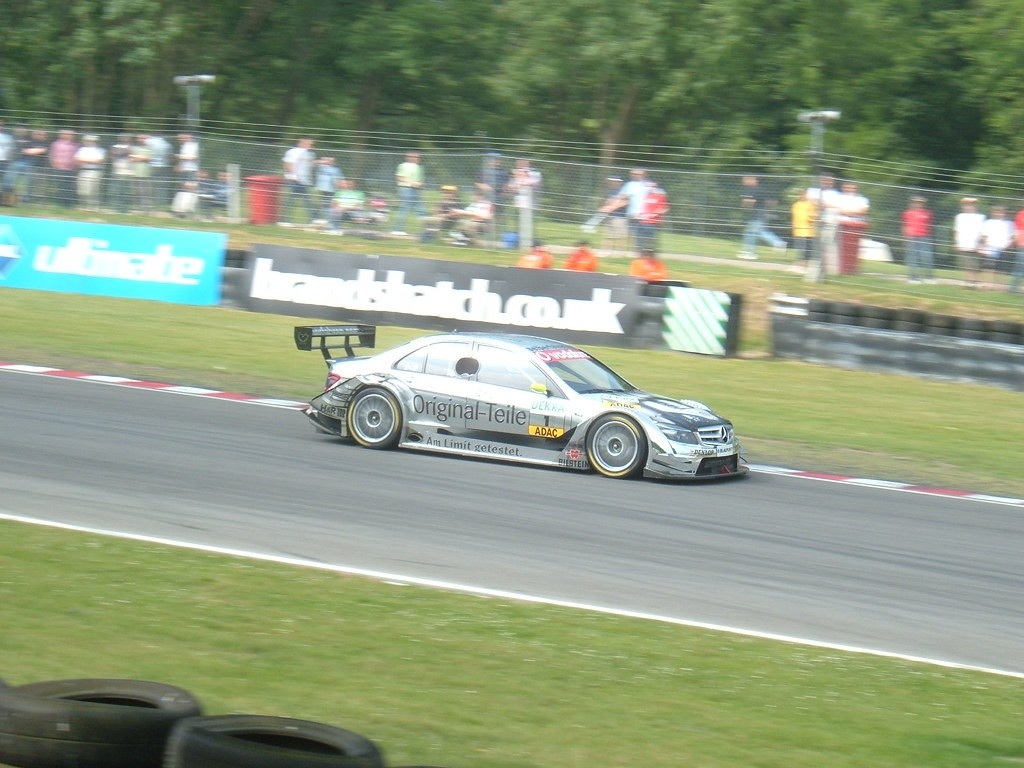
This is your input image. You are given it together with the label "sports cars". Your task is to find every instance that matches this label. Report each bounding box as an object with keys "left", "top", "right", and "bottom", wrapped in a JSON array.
[{"left": 294, "top": 324, "right": 749, "bottom": 481}]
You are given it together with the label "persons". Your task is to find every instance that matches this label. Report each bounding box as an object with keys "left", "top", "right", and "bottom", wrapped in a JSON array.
[
  {"left": 789, "top": 171, "right": 871, "bottom": 276},
  {"left": 900, "top": 196, "right": 941, "bottom": 285},
  {"left": 737, "top": 175, "right": 788, "bottom": 260},
  {"left": 1005, "top": 210, "right": 1024, "bottom": 293},
  {"left": 953, "top": 198, "right": 1015, "bottom": 288},
  {"left": 516, "top": 239, "right": 669, "bottom": 280},
  {"left": 276, "top": 138, "right": 431, "bottom": 237},
  {"left": 597, "top": 167, "right": 669, "bottom": 259},
  {"left": 421, "top": 153, "right": 542, "bottom": 248},
  {"left": 0, "top": 119, "right": 238, "bottom": 221}
]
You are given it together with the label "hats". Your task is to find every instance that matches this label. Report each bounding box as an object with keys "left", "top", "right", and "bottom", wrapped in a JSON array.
[{"left": 960, "top": 196, "right": 979, "bottom": 206}]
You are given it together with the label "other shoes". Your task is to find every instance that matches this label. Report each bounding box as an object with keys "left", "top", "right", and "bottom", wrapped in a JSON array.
[
  {"left": 738, "top": 252, "right": 760, "bottom": 261},
  {"left": 391, "top": 229, "right": 409, "bottom": 238}
]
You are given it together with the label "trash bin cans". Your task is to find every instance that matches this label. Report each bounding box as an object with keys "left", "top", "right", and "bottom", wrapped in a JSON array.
[
  {"left": 837, "top": 221, "right": 870, "bottom": 274},
  {"left": 244, "top": 176, "right": 285, "bottom": 225}
]
